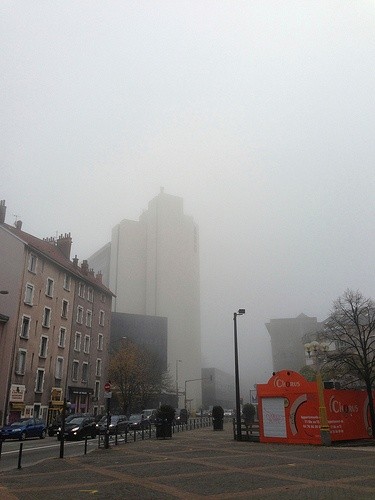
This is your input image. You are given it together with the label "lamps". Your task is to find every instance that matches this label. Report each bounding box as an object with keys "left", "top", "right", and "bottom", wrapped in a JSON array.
[
  {"left": 273, "top": 372, "right": 279, "bottom": 376},
  {"left": 287, "top": 371, "right": 294, "bottom": 375}
]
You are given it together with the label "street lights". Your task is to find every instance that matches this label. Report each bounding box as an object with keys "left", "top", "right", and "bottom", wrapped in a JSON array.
[
  {"left": 232, "top": 308, "right": 246, "bottom": 441},
  {"left": 175, "top": 359, "right": 182, "bottom": 421},
  {"left": 304, "top": 340, "right": 331, "bottom": 447}
]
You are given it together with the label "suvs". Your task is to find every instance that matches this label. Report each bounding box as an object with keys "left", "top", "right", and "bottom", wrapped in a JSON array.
[{"left": 139, "top": 409, "right": 158, "bottom": 422}]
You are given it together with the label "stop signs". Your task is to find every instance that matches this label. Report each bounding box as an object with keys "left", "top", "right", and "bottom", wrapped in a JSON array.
[{"left": 105, "top": 383, "right": 111, "bottom": 392}]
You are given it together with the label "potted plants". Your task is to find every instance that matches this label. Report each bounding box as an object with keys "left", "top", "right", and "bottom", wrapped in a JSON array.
[
  {"left": 242, "top": 403, "right": 255, "bottom": 424},
  {"left": 212, "top": 406, "right": 224, "bottom": 430},
  {"left": 155, "top": 405, "right": 176, "bottom": 437}
]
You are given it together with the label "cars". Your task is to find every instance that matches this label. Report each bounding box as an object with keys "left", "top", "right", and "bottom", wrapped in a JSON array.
[
  {"left": 48, "top": 414, "right": 73, "bottom": 437},
  {"left": 194, "top": 408, "right": 236, "bottom": 417},
  {"left": 57, "top": 416, "right": 96, "bottom": 441},
  {"left": 96, "top": 414, "right": 129, "bottom": 435},
  {"left": 126, "top": 413, "right": 150, "bottom": 431},
  {"left": 0, "top": 418, "right": 47, "bottom": 441}
]
